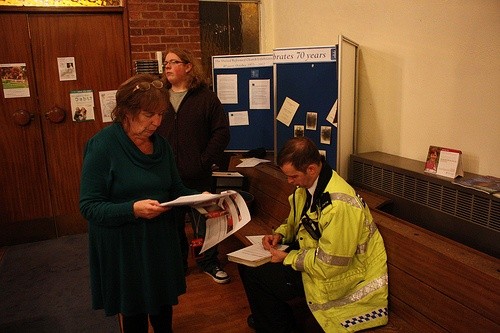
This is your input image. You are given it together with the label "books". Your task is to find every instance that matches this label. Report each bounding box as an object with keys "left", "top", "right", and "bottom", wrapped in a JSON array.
[
  {"left": 228, "top": 242, "right": 284, "bottom": 267},
  {"left": 450, "top": 175, "right": 500, "bottom": 195}
]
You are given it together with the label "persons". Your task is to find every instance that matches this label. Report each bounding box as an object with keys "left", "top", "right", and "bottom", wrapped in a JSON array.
[
  {"left": 156, "top": 48, "right": 231, "bottom": 284},
  {"left": 237, "top": 137, "right": 389, "bottom": 333},
  {"left": 78, "top": 74, "right": 213, "bottom": 333},
  {"left": 425, "top": 147, "right": 439, "bottom": 172}
]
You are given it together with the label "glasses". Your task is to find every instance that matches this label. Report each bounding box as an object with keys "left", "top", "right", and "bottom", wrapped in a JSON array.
[
  {"left": 119, "top": 80, "right": 164, "bottom": 108},
  {"left": 161, "top": 59, "right": 188, "bottom": 67}
]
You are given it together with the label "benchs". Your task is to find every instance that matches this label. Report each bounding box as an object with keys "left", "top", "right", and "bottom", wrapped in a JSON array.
[{"left": 216, "top": 155, "right": 500, "bottom": 333}]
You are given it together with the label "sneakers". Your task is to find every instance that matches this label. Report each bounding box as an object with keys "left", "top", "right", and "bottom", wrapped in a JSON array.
[{"left": 199, "top": 253, "right": 230, "bottom": 284}]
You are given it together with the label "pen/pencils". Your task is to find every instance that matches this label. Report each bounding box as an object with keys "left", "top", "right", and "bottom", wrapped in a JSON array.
[{"left": 271, "top": 224, "right": 276, "bottom": 237}]
